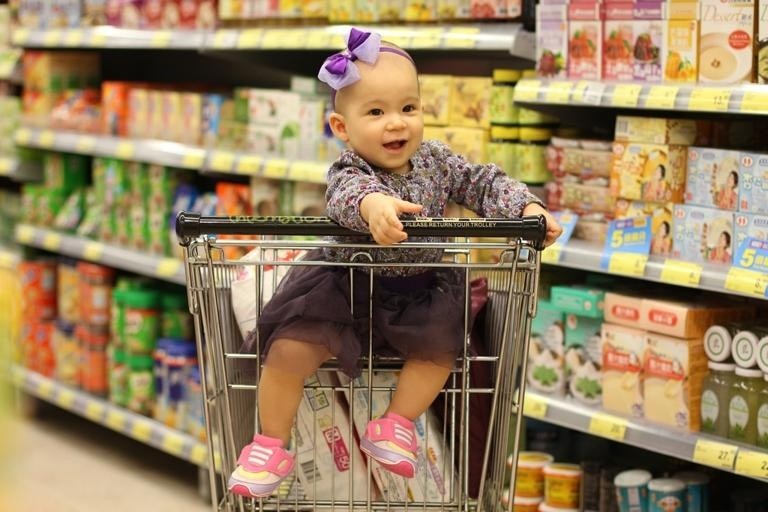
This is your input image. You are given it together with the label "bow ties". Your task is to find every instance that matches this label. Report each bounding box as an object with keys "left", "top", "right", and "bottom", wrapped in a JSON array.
[{"left": 226, "top": 434, "right": 297, "bottom": 498}]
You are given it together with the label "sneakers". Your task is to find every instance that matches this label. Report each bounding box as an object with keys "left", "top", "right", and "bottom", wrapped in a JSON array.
[{"left": 358, "top": 412, "right": 420, "bottom": 479}]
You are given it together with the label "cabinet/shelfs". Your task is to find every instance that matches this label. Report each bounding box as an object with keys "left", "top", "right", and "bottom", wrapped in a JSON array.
[
  {"left": 504, "top": 73, "right": 767, "bottom": 512},
  {"left": 0, "top": 17, "right": 534, "bottom": 503}
]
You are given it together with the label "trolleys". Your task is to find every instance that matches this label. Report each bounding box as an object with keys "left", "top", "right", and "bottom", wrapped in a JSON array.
[{"left": 174, "top": 208, "right": 546, "bottom": 512}]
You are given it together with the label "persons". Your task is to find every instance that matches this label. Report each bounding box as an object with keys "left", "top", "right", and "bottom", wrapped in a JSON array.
[
  {"left": 710, "top": 230, "right": 732, "bottom": 264},
  {"left": 718, "top": 171, "right": 738, "bottom": 210},
  {"left": 226, "top": 29, "right": 563, "bottom": 498},
  {"left": 650, "top": 220, "right": 671, "bottom": 255},
  {"left": 644, "top": 164, "right": 666, "bottom": 202}
]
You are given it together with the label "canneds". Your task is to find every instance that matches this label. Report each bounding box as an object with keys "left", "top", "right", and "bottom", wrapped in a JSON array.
[{"left": 700, "top": 317, "right": 768, "bottom": 450}]
